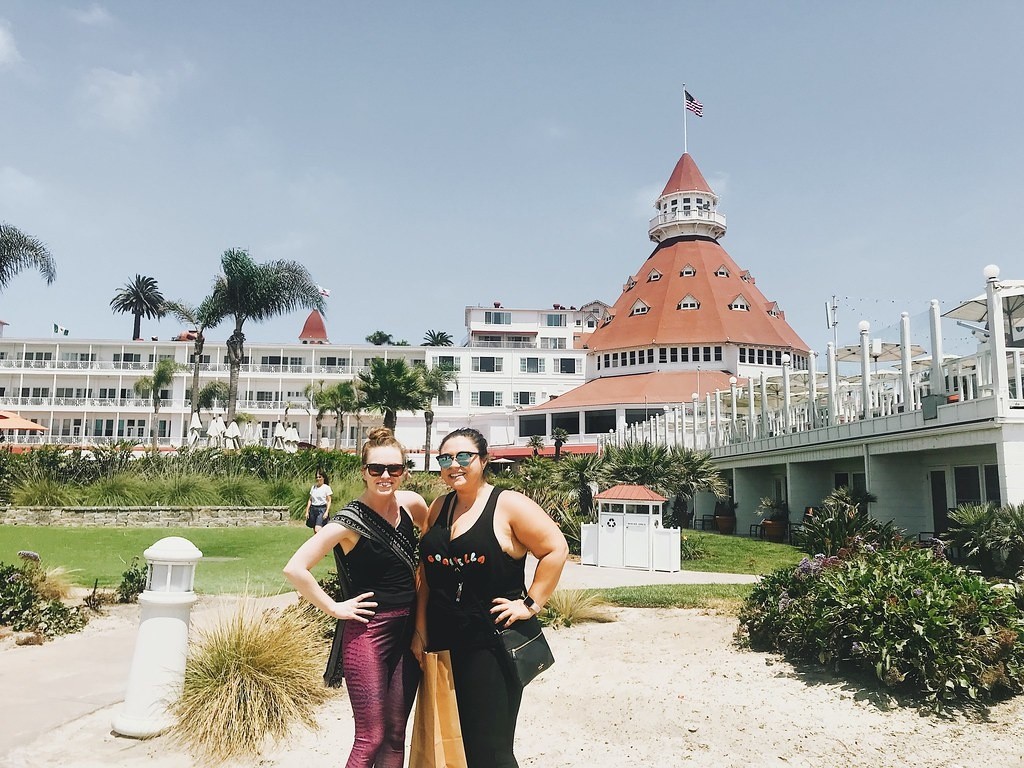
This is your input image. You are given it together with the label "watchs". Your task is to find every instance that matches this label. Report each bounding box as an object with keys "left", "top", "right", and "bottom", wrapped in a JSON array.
[{"left": 523, "top": 596, "right": 542, "bottom": 614}]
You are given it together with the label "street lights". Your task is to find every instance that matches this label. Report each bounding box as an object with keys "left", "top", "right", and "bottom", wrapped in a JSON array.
[
  {"left": 697, "top": 363, "right": 701, "bottom": 395},
  {"left": 645, "top": 395, "right": 648, "bottom": 421}
]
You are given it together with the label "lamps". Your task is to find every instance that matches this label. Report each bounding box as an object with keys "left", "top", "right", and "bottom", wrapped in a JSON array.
[{"left": 143, "top": 536, "right": 203, "bottom": 594}]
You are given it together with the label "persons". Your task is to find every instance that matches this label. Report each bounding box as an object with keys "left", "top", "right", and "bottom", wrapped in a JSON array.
[
  {"left": 305, "top": 471, "right": 334, "bottom": 535},
  {"left": 410, "top": 429, "right": 569, "bottom": 768},
  {"left": 283, "top": 427, "right": 430, "bottom": 768}
]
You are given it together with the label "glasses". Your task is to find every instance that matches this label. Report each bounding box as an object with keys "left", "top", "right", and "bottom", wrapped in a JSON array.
[
  {"left": 364, "top": 463, "right": 405, "bottom": 477},
  {"left": 436, "top": 451, "right": 483, "bottom": 469},
  {"left": 316, "top": 476, "right": 323, "bottom": 479}
]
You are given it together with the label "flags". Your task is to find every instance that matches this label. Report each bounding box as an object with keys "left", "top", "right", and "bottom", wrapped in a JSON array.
[
  {"left": 685, "top": 90, "right": 704, "bottom": 117},
  {"left": 318, "top": 286, "right": 331, "bottom": 297},
  {"left": 53, "top": 322, "right": 70, "bottom": 336}
]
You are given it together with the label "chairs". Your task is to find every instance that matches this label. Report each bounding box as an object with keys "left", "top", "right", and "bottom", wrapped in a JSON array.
[
  {"left": 789, "top": 507, "right": 823, "bottom": 546},
  {"left": 693, "top": 514, "right": 714, "bottom": 530},
  {"left": 750, "top": 518, "right": 765, "bottom": 538}
]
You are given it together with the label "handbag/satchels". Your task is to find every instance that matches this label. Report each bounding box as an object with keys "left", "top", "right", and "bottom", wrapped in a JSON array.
[
  {"left": 494, "top": 612, "right": 555, "bottom": 687},
  {"left": 305, "top": 508, "right": 316, "bottom": 528},
  {"left": 408, "top": 649, "right": 468, "bottom": 768}
]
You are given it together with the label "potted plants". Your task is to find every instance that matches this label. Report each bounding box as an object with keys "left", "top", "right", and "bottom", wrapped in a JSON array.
[
  {"left": 754, "top": 497, "right": 789, "bottom": 544},
  {"left": 714, "top": 500, "right": 738, "bottom": 536}
]
